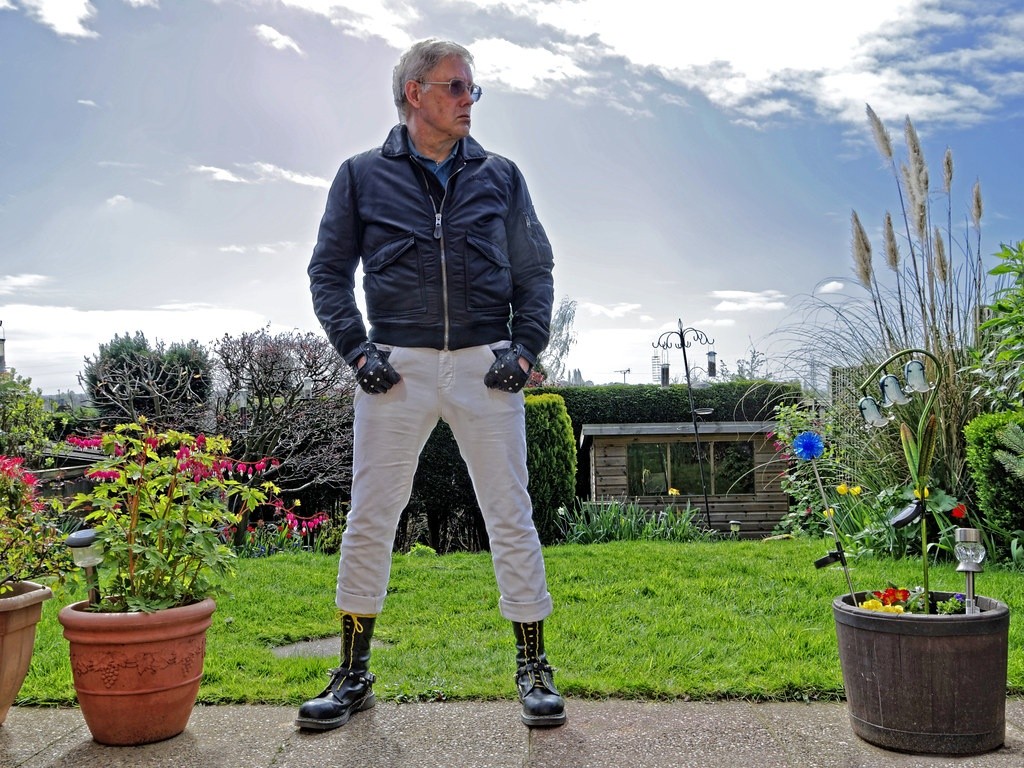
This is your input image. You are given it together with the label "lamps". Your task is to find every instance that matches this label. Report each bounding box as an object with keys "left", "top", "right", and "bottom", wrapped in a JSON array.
[
  {"left": 954, "top": 527, "right": 987, "bottom": 615},
  {"left": 64, "top": 528, "right": 105, "bottom": 606},
  {"left": 729, "top": 519, "right": 742, "bottom": 540}
]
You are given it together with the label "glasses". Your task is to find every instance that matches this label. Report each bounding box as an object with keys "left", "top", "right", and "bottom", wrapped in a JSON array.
[{"left": 422, "top": 79, "right": 482, "bottom": 103}]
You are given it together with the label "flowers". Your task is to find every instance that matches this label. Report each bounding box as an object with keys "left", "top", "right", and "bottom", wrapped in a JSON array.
[
  {"left": 67, "top": 416, "right": 331, "bottom": 612},
  {"left": 861, "top": 580, "right": 965, "bottom": 615},
  {"left": 0, "top": 449, "right": 74, "bottom": 596}
]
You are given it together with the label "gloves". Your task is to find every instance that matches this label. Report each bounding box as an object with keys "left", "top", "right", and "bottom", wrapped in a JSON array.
[
  {"left": 349, "top": 344, "right": 401, "bottom": 395},
  {"left": 484, "top": 344, "right": 537, "bottom": 393}
]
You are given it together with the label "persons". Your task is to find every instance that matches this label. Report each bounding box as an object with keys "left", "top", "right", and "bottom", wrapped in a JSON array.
[{"left": 294, "top": 39, "right": 567, "bottom": 730}]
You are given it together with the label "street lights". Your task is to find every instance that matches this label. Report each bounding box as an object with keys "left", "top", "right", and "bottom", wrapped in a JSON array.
[{"left": 651, "top": 319, "right": 720, "bottom": 532}]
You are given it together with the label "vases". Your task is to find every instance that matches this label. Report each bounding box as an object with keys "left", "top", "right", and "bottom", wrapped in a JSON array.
[
  {"left": 833, "top": 590, "right": 1010, "bottom": 753},
  {"left": 0, "top": 580, "right": 52, "bottom": 724},
  {"left": 58, "top": 594, "right": 215, "bottom": 746}
]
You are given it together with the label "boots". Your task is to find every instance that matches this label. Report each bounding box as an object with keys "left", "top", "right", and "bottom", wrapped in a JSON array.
[
  {"left": 512, "top": 620, "right": 566, "bottom": 726},
  {"left": 295, "top": 611, "right": 377, "bottom": 730}
]
